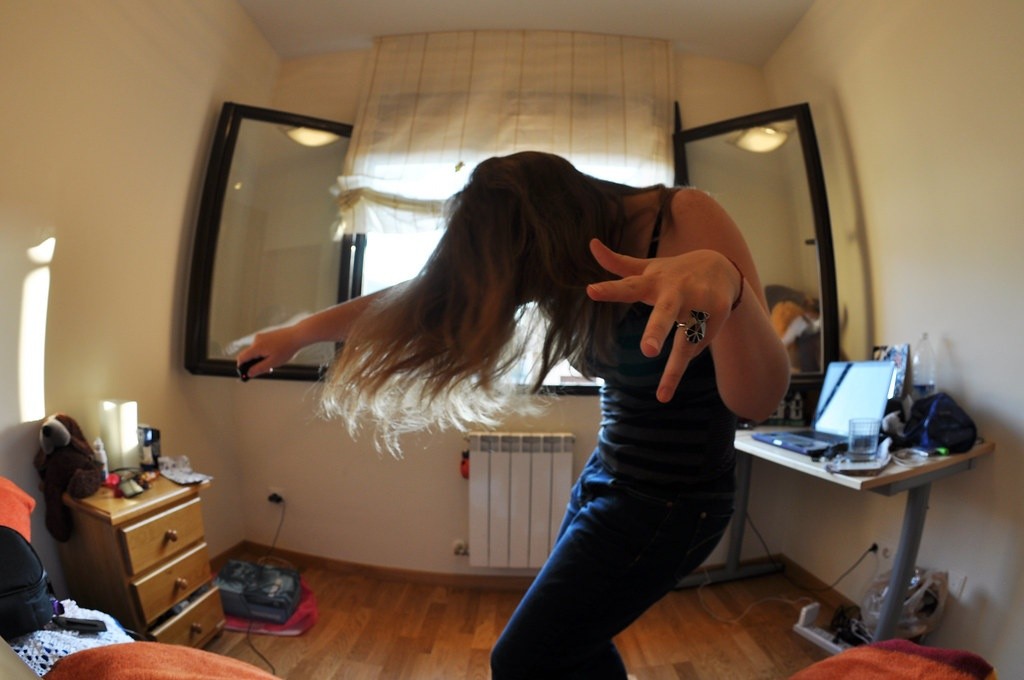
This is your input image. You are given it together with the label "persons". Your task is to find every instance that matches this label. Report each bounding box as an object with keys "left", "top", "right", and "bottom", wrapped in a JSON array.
[{"left": 235, "top": 151, "right": 792, "bottom": 680}]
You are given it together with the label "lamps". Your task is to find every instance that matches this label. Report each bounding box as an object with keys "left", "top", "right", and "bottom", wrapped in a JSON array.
[
  {"left": 98, "top": 398, "right": 139, "bottom": 470},
  {"left": 732, "top": 124, "right": 789, "bottom": 154},
  {"left": 284, "top": 126, "right": 340, "bottom": 149}
]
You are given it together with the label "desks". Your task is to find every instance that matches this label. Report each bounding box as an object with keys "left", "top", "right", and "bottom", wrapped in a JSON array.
[{"left": 670, "top": 420, "right": 995, "bottom": 655}]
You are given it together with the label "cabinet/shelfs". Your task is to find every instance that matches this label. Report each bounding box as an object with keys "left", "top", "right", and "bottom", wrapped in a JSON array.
[{"left": 61, "top": 468, "right": 227, "bottom": 650}]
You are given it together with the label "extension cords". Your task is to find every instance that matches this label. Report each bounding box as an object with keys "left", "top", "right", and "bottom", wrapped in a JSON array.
[{"left": 794, "top": 621, "right": 854, "bottom": 655}]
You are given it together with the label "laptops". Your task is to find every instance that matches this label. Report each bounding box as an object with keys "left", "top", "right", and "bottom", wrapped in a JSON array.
[{"left": 752, "top": 360, "right": 896, "bottom": 456}]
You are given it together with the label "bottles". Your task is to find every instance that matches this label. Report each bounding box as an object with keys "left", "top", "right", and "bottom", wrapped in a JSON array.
[
  {"left": 91, "top": 436, "right": 108, "bottom": 479},
  {"left": 912, "top": 333, "right": 937, "bottom": 401}
]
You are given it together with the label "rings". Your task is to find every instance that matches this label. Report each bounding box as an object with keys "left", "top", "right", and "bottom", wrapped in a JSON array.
[{"left": 677, "top": 309, "right": 711, "bottom": 344}]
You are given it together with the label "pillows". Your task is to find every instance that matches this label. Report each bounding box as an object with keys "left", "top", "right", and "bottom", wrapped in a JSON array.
[{"left": 41, "top": 640, "right": 285, "bottom": 680}]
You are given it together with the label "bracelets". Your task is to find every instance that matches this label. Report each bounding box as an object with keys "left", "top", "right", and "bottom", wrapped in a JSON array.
[{"left": 724, "top": 256, "right": 743, "bottom": 312}]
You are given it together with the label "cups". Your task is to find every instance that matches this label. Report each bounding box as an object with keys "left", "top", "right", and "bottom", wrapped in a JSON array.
[{"left": 849, "top": 418, "right": 880, "bottom": 461}]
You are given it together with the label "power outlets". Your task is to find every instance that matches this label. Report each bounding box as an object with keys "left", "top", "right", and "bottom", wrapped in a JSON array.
[{"left": 267, "top": 488, "right": 289, "bottom": 511}]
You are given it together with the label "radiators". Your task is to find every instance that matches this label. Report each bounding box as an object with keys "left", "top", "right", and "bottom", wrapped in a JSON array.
[{"left": 467, "top": 431, "right": 575, "bottom": 570}]
